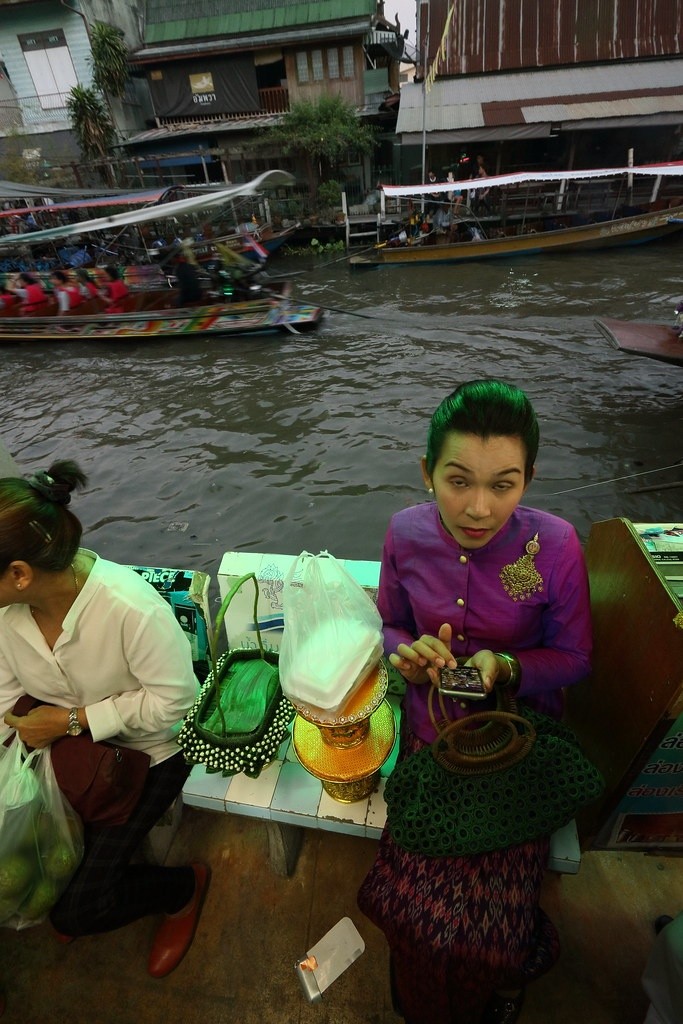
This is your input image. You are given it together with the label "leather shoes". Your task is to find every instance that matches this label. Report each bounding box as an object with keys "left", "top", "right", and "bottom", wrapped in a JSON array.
[
  {"left": 148, "top": 861, "right": 211, "bottom": 978},
  {"left": 55, "top": 934, "right": 74, "bottom": 945}
]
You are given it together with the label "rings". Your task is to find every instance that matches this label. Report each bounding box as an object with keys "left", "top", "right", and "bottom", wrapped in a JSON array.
[{"left": 452, "top": 697, "right": 458, "bottom": 702}]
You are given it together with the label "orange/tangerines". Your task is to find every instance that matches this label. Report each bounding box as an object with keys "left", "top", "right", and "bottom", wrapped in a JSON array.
[{"left": 0, "top": 805, "right": 83, "bottom": 923}]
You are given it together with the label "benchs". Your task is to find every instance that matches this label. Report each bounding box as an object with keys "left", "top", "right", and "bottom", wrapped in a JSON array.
[{"left": 180, "top": 549, "right": 582, "bottom": 879}]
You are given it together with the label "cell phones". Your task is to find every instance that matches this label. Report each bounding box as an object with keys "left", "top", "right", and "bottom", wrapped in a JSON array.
[{"left": 438, "top": 666, "right": 487, "bottom": 700}]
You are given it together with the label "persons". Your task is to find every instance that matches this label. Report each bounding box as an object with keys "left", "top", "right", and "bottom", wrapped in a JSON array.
[
  {"left": 428, "top": 152, "right": 492, "bottom": 217},
  {"left": 0, "top": 200, "right": 260, "bottom": 317},
  {"left": 432, "top": 201, "right": 458, "bottom": 236},
  {"left": 356, "top": 379, "right": 593, "bottom": 1024},
  {"left": 0, "top": 459, "right": 208, "bottom": 977}
]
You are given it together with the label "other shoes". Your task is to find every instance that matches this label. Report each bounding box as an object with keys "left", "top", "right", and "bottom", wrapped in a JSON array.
[{"left": 480, "top": 988, "right": 524, "bottom": 1024}]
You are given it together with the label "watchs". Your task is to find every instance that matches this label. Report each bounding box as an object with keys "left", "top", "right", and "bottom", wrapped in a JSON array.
[{"left": 66, "top": 707, "right": 83, "bottom": 738}]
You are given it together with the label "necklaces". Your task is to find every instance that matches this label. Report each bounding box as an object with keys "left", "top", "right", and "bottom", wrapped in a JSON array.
[{"left": 31, "top": 562, "right": 79, "bottom": 615}]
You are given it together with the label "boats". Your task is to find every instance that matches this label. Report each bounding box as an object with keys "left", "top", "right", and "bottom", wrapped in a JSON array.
[
  {"left": 370, "top": 158, "right": 682, "bottom": 264},
  {"left": 0, "top": 169, "right": 326, "bottom": 340}
]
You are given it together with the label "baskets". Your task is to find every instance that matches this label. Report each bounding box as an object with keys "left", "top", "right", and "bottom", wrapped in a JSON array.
[{"left": 176, "top": 572, "right": 296, "bottom": 779}]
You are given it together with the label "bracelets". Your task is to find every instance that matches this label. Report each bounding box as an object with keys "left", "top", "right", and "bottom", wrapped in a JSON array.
[{"left": 495, "top": 651, "right": 519, "bottom": 690}]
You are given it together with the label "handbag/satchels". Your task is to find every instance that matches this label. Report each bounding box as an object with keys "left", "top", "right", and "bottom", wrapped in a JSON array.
[
  {"left": 382, "top": 684, "right": 606, "bottom": 856},
  {"left": 9, "top": 696, "right": 151, "bottom": 827},
  {"left": 278, "top": 551, "right": 385, "bottom": 722},
  {"left": 0, "top": 730, "right": 84, "bottom": 931}
]
